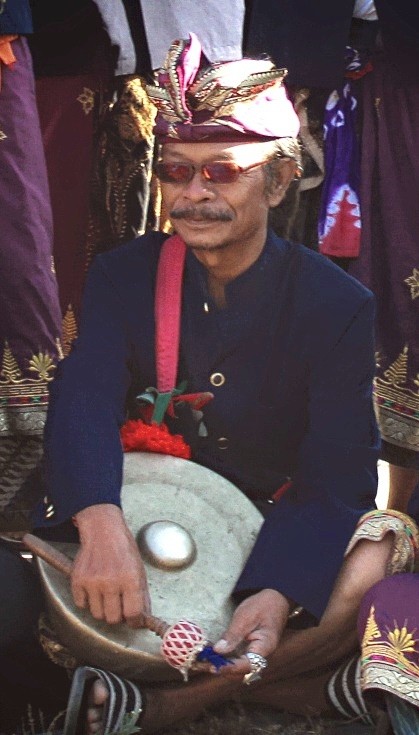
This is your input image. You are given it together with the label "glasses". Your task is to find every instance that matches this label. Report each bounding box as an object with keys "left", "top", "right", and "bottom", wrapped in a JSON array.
[{"left": 152, "top": 153, "right": 286, "bottom": 187}]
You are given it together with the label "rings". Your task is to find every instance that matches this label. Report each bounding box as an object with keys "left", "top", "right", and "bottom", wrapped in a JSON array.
[{"left": 242, "top": 650, "right": 269, "bottom": 690}]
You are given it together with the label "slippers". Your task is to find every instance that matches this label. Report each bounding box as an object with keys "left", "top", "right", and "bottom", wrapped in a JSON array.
[
  {"left": 327, "top": 652, "right": 376, "bottom": 727},
  {"left": 63, "top": 666, "right": 144, "bottom": 735}
]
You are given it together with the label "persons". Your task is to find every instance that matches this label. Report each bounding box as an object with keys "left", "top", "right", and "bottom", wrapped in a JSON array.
[
  {"left": 0, "top": 0, "right": 418, "bottom": 398},
  {"left": 1, "top": 27, "right": 418, "bottom": 735}
]
